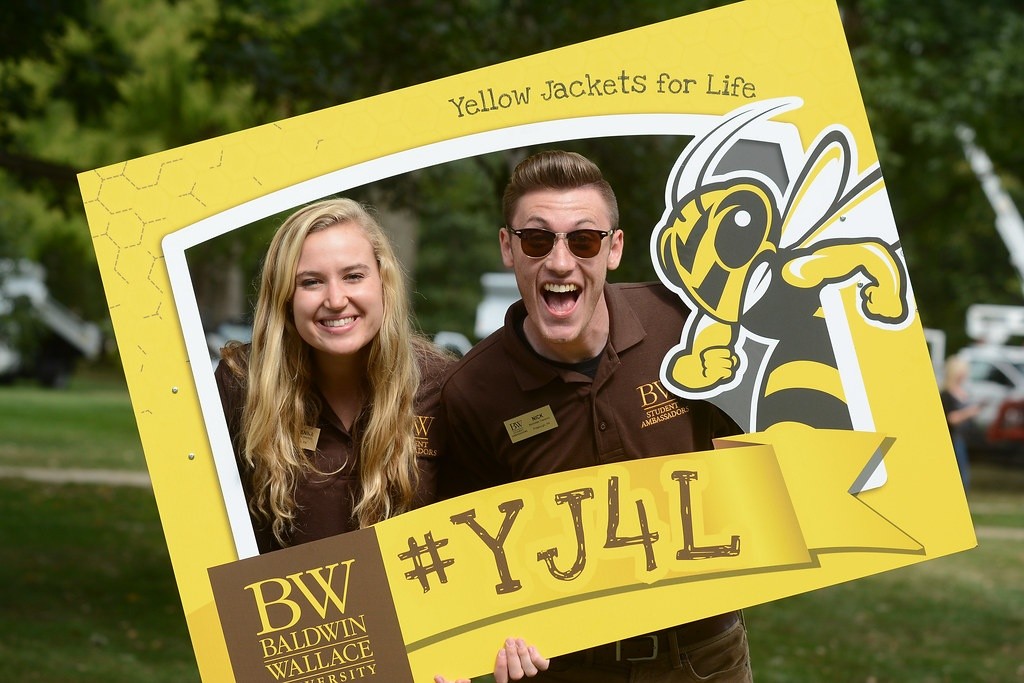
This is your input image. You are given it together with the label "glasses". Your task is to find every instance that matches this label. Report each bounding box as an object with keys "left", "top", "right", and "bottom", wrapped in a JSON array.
[{"left": 505, "top": 222, "right": 613, "bottom": 259}]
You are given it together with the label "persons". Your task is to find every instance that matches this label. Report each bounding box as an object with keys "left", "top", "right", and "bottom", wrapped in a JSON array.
[
  {"left": 938, "top": 355, "right": 992, "bottom": 491},
  {"left": 424, "top": 151, "right": 753, "bottom": 683},
  {"left": 213, "top": 197, "right": 474, "bottom": 683}
]
